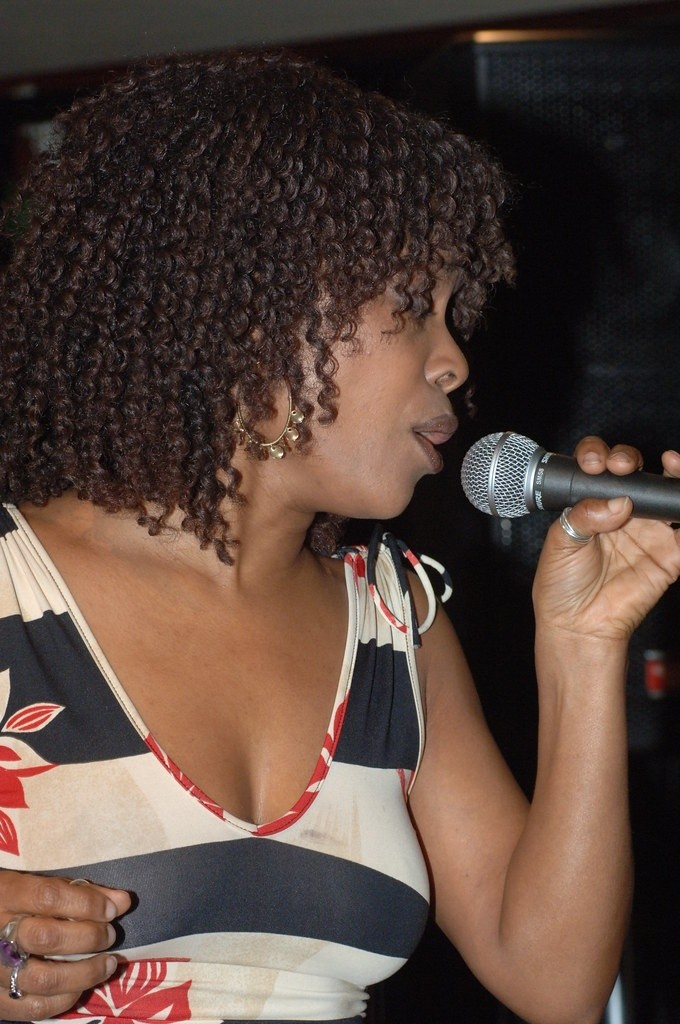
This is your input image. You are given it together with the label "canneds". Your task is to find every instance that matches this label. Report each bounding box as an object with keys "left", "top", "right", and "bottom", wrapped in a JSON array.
[{"left": 643, "top": 649, "right": 667, "bottom": 700}]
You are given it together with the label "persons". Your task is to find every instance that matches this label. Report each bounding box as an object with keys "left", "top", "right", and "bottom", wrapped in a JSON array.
[{"left": 0, "top": 40, "right": 680, "bottom": 1024}]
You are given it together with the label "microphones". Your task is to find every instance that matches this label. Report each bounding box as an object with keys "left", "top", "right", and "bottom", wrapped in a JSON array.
[{"left": 461, "top": 432, "right": 680, "bottom": 523}]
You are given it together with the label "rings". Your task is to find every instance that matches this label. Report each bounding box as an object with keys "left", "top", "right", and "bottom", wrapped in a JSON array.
[
  {"left": 559, "top": 506, "right": 594, "bottom": 544},
  {"left": 0, "top": 910, "right": 35, "bottom": 1001}
]
